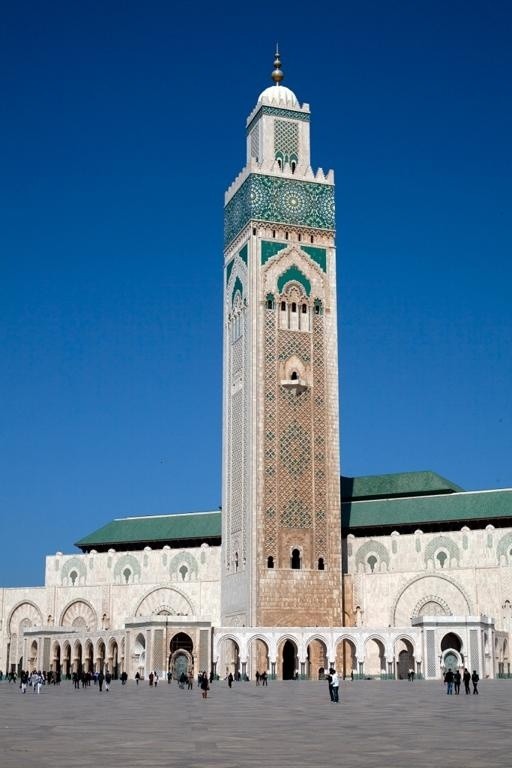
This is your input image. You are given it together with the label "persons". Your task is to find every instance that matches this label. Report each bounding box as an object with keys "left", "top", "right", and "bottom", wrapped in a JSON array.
[
  {"left": 454, "top": 670, "right": 461, "bottom": 695},
  {"left": 408, "top": 664, "right": 414, "bottom": 682},
  {"left": 444, "top": 669, "right": 454, "bottom": 696},
  {"left": 472, "top": 671, "right": 479, "bottom": 695},
  {"left": 329, "top": 670, "right": 339, "bottom": 704},
  {"left": 326, "top": 668, "right": 334, "bottom": 701},
  {"left": 463, "top": 669, "right": 471, "bottom": 694},
  {"left": 0, "top": 670, "right": 268, "bottom": 699}
]
